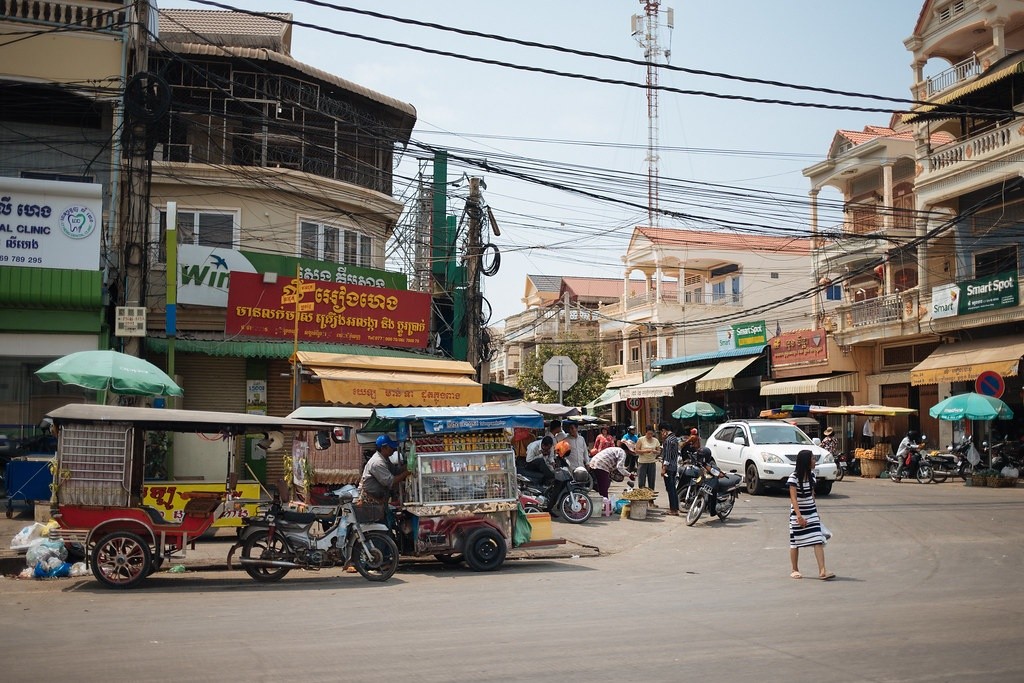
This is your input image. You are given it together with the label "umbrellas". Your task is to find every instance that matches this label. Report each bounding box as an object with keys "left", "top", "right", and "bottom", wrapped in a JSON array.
[
  {"left": 672, "top": 401, "right": 727, "bottom": 437},
  {"left": 34, "top": 348, "right": 184, "bottom": 405},
  {"left": 930, "top": 392, "right": 1014, "bottom": 436}
]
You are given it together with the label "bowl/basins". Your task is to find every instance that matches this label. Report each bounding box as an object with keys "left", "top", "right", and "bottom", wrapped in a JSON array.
[{"left": 422, "top": 462, "right": 429, "bottom": 467}]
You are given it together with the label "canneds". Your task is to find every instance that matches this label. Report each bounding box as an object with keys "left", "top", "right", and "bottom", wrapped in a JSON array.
[{"left": 430, "top": 459, "right": 500, "bottom": 473}]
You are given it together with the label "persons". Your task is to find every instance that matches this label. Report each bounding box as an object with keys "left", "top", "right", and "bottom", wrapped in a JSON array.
[
  {"left": 819, "top": 428, "right": 838, "bottom": 456},
  {"left": 896, "top": 431, "right": 926, "bottom": 483},
  {"left": 591, "top": 426, "right": 661, "bottom": 508},
  {"left": 658, "top": 421, "right": 679, "bottom": 516},
  {"left": 354, "top": 435, "right": 410, "bottom": 568},
  {"left": 863, "top": 417, "right": 876, "bottom": 448},
  {"left": 787, "top": 450, "right": 835, "bottom": 580},
  {"left": 525, "top": 421, "right": 590, "bottom": 513},
  {"left": 684, "top": 429, "right": 700, "bottom": 462}
]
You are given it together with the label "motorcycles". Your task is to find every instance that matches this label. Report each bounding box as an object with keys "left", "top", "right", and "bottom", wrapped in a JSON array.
[
  {"left": 686, "top": 462, "right": 744, "bottom": 526},
  {"left": 927, "top": 435, "right": 988, "bottom": 483},
  {"left": 886, "top": 435, "right": 934, "bottom": 484},
  {"left": 516, "top": 453, "right": 595, "bottom": 524},
  {"left": 673, "top": 451, "right": 702, "bottom": 513},
  {"left": 227, "top": 483, "right": 399, "bottom": 582},
  {"left": 957, "top": 434, "right": 1019, "bottom": 481},
  {"left": 833, "top": 452, "right": 848, "bottom": 481}
]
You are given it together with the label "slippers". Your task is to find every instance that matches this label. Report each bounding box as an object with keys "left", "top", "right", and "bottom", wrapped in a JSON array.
[
  {"left": 791, "top": 571, "right": 801, "bottom": 578},
  {"left": 820, "top": 571, "right": 836, "bottom": 579}
]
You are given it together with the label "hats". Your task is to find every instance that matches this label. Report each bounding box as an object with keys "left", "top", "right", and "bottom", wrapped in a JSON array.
[
  {"left": 617, "top": 439, "right": 636, "bottom": 453},
  {"left": 376, "top": 434, "right": 398, "bottom": 448},
  {"left": 824, "top": 426, "right": 833, "bottom": 435}
]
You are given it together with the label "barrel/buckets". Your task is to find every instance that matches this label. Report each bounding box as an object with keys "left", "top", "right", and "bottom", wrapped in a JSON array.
[
  {"left": 616, "top": 499, "right": 631, "bottom": 513},
  {"left": 629, "top": 500, "right": 649, "bottom": 520},
  {"left": 581, "top": 491, "right": 603, "bottom": 517}
]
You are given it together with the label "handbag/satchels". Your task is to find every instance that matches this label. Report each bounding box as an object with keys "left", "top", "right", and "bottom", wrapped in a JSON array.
[
  {"left": 821, "top": 523, "right": 832, "bottom": 543},
  {"left": 906, "top": 453, "right": 912, "bottom": 464}
]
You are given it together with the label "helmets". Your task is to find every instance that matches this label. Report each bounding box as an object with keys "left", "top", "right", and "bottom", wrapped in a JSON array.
[
  {"left": 573, "top": 466, "right": 588, "bottom": 481},
  {"left": 628, "top": 425, "right": 636, "bottom": 432},
  {"left": 908, "top": 431, "right": 917, "bottom": 441},
  {"left": 691, "top": 428, "right": 698, "bottom": 434},
  {"left": 697, "top": 446, "right": 711, "bottom": 462}
]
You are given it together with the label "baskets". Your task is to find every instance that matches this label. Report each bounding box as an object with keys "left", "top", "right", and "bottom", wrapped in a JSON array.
[{"left": 352, "top": 503, "right": 384, "bottom": 522}]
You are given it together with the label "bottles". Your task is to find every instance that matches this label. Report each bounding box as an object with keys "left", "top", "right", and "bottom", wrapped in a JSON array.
[
  {"left": 623, "top": 489, "right": 627, "bottom": 496},
  {"left": 453, "top": 463, "right": 467, "bottom": 472},
  {"left": 443, "top": 433, "right": 506, "bottom": 451},
  {"left": 485, "top": 460, "right": 504, "bottom": 472},
  {"left": 475, "top": 464, "right": 479, "bottom": 472},
  {"left": 409, "top": 436, "right": 444, "bottom": 451}
]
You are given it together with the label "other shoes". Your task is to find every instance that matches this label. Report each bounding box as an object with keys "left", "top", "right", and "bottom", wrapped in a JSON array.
[
  {"left": 661, "top": 509, "right": 679, "bottom": 516},
  {"left": 648, "top": 503, "right": 659, "bottom": 509},
  {"left": 543, "top": 507, "right": 558, "bottom": 518},
  {"left": 895, "top": 479, "right": 903, "bottom": 483}
]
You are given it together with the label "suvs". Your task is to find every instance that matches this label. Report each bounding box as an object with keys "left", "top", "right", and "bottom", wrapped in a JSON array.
[{"left": 705, "top": 418, "right": 837, "bottom": 496}]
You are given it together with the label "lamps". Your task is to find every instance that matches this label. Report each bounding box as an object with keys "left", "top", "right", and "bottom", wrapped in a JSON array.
[{"left": 263, "top": 272, "right": 277, "bottom": 283}]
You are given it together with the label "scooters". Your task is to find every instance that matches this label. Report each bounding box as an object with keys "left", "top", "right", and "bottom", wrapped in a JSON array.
[{"left": 323, "top": 501, "right": 518, "bottom": 571}]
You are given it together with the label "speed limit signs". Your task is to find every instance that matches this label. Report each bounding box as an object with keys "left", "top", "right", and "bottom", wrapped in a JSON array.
[{"left": 626, "top": 398, "right": 643, "bottom": 412}]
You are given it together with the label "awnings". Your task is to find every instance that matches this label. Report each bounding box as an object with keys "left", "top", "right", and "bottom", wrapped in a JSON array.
[
  {"left": 760, "top": 371, "right": 859, "bottom": 395},
  {"left": 910, "top": 335, "right": 1024, "bottom": 385},
  {"left": 620, "top": 364, "right": 713, "bottom": 398},
  {"left": 583, "top": 389, "right": 626, "bottom": 408},
  {"left": 308, "top": 366, "right": 482, "bottom": 407},
  {"left": 696, "top": 354, "right": 760, "bottom": 392}
]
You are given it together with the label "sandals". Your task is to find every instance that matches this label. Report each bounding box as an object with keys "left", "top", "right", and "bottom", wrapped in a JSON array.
[{"left": 347, "top": 566, "right": 357, "bottom": 572}]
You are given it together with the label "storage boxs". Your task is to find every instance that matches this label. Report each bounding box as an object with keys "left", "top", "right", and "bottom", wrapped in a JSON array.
[
  {"left": 525, "top": 512, "right": 552, "bottom": 540},
  {"left": 971, "top": 477, "right": 1018, "bottom": 488}
]
